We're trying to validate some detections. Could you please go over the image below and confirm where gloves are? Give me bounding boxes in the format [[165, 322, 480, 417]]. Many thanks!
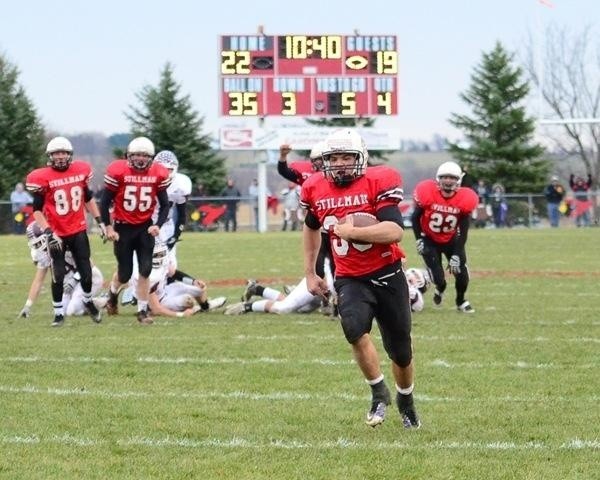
[[43, 231, 65, 256], [98, 222, 108, 244], [415, 238, 432, 257], [445, 254, 463, 276]]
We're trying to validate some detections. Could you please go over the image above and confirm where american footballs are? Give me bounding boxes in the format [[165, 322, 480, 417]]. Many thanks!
[[338, 213, 380, 227]]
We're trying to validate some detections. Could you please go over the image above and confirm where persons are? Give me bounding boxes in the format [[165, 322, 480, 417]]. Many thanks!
[[25, 136, 109, 328], [98, 135, 172, 325], [11, 183, 108, 318], [276, 140, 333, 279], [410, 161, 479, 315], [121, 269, 226, 318], [190, 177, 303, 232], [223, 258, 433, 318], [543, 173, 594, 228], [297, 127, 423, 430], [148, 149, 193, 278], [468, 177, 509, 229]]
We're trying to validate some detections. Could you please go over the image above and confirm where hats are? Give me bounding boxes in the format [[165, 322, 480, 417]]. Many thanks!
[[319, 128, 369, 179]]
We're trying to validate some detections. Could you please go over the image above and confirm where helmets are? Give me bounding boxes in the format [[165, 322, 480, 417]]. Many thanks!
[[434, 161, 465, 194], [45, 136, 74, 171], [406, 263, 430, 313], [24, 221, 48, 262], [153, 149, 179, 186], [308, 145, 321, 161], [125, 135, 157, 172], [150, 238, 170, 267]]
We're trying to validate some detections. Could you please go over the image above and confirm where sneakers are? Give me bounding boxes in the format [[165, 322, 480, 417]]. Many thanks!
[[282, 283, 295, 294], [240, 279, 258, 303], [432, 287, 443, 307], [81, 299, 101, 323], [222, 301, 247, 315], [395, 390, 421, 431], [135, 309, 153, 326], [48, 313, 65, 326], [17, 306, 30, 320], [106, 292, 119, 315], [364, 380, 392, 428], [456, 300, 475, 314], [202, 296, 227, 313]]
